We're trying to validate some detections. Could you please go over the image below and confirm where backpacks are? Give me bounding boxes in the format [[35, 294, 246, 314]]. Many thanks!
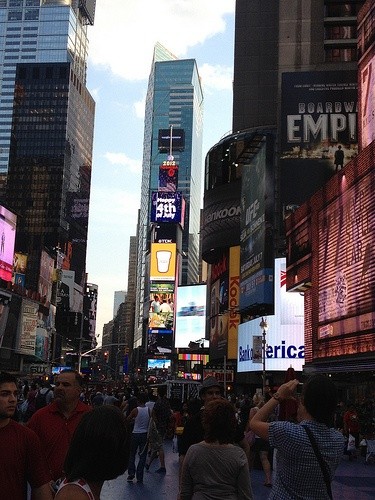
[[36, 388, 51, 409]]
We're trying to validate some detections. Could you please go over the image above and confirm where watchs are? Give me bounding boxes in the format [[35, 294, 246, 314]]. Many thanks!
[[273, 393, 281, 402]]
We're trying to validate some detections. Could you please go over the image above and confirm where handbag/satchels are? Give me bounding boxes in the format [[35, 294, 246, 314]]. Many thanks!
[[250, 433, 262, 454], [21, 398, 30, 416]]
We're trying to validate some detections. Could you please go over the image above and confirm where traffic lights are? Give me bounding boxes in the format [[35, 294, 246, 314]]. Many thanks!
[[104, 353, 108, 360], [45, 376, 49, 382], [125, 347, 130, 354]]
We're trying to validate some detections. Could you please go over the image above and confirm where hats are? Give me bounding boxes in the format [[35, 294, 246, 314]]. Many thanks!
[[198, 376, 223, 398]]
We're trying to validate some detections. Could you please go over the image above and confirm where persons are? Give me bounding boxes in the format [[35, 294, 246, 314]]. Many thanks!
[[144, 387, 176, 473], [249, 373, 346, 500], [11, 375, 55, 424], [335, 398, 360, 454], [334, 144, 344, 171], [55, 406, 129, 500], [159, 298, 174, 312], [80, 374, 174, 415], [228, 392, 281, 487], [151, 295, 161, 312], [25, 369, 91, 497], [126, 395, 154, 482], [1, 232, 5, 255], [178, 375, 251, 471], [177, 400, 254, 500], [51, 269, 73, 310], [0, 370, 53, 500]]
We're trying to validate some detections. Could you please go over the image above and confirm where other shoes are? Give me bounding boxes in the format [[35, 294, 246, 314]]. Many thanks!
[[127, 475, 135, 482], [264, 483, 273, 488], [144, 463, 150, 470], [157, 467, 166, 474], [137, 473, 144, 482]]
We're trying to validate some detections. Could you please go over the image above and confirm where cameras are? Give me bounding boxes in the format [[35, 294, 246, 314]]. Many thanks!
[[294, 383, 304, 395]]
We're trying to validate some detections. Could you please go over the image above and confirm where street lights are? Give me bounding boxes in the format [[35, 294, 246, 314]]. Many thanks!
[[259, 317, 270, 396]]
